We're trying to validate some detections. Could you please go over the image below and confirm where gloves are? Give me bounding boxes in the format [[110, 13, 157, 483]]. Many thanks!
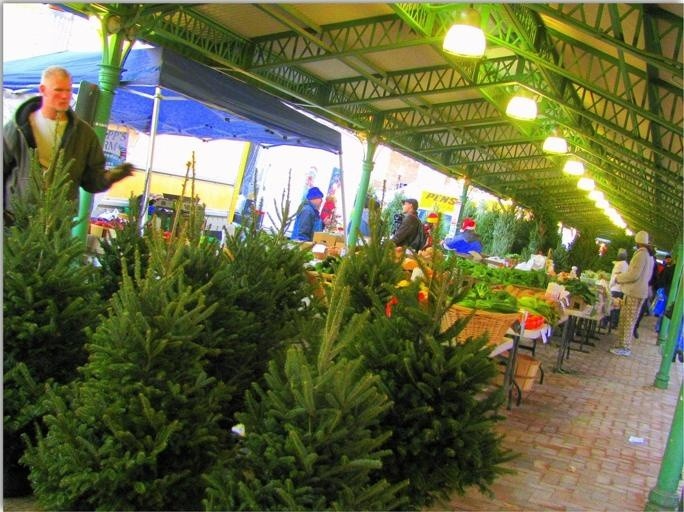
[[107, 164, 134, 184]]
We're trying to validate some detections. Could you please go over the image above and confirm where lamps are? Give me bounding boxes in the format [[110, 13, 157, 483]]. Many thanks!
[[438, 4, 595, 191]]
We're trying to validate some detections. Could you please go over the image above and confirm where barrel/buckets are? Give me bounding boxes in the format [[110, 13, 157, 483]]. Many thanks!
[[487, 353, 542, 399]]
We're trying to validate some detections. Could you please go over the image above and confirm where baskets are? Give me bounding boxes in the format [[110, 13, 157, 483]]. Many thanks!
[[508, 284, 547, 300], [487, 283, 507, 293], [442, 299, 524, 348]]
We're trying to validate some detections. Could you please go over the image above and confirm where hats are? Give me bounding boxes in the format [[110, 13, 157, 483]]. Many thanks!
[[401, 198, 418, 211], [460, 217, 475, 233], [617, 248, 627, 259], [306, 187, 323, 200], [635, 230, 648, 245]]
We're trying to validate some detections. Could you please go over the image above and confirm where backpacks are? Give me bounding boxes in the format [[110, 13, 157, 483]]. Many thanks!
[[409, 216, 427, 251]]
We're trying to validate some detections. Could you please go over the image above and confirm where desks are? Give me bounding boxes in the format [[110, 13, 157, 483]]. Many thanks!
[[436, 294, 622, 411]]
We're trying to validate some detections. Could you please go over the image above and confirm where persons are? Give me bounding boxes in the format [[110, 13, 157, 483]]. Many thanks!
[[391, 198, 424, 253], [347, 200, 382, 247], [290, 186, 325, 241], [2, 63, 135, 226], [532, 248, 547, 270], [442, 227, 482, 254], [600, 230, 675, 357]]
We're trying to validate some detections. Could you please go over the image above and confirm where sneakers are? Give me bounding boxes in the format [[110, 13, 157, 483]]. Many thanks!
[[610, 344, 631, 358]]
